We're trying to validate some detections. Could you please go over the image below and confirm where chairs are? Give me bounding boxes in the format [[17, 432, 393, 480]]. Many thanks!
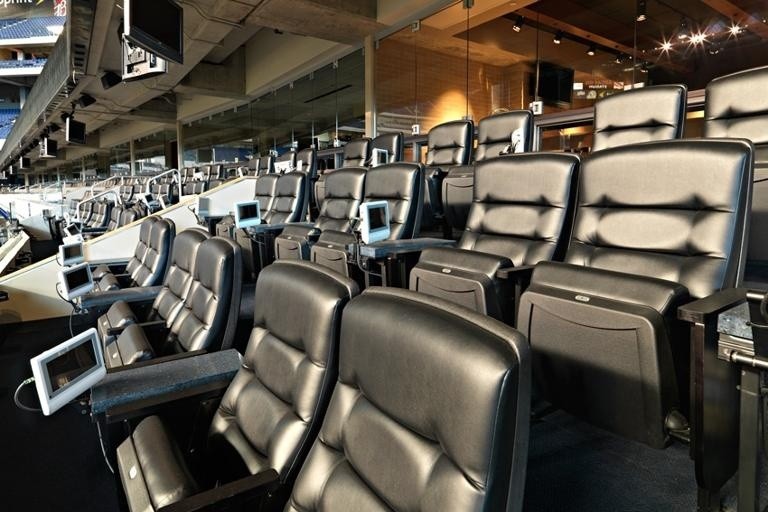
[[281, 152, 296, 172], [296, 148, 318, 180], [367, 130, 404, 166], [341, 137, 373, 167], [95, 214, 163, 281], [115, 257, 361, 511], [99, 218, 177, 291], [217, 173, 279, 240], [426, 120, 475, 166], [68, 160, 236, 230], [518, 136, 755, 512], [95, 226, 211, 345], [408, 153, 582, 331], [267, 166, 368, 263], [259, 154, 274, 176], [704, 65, 768, 262], [310, 160, 426, 285], [248, 158, 260, 175], [167, 283, 534, 512], [106, 234, 243, 376], [473, 109, 534, 161], [590, 84, 687, 152], [233, 170, 310, 277]]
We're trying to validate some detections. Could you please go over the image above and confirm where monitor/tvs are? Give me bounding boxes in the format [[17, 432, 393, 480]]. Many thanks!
[[44, 138, 58, 159], [537, 60, 574, 104], [197, 197, 211, 215], [31, 328, 106, 416], [66, 117, 86, 144], [62, 262, 94, 301], [373, 149, 389, 165], [124, 0, 184, 67], [235, 201, 261, 228], [360, 203, 390, 243], [144, 194, 154, 202], [65, 223, 80, 235], [121, 33, 168, 82], [61, 243, 82, 264]]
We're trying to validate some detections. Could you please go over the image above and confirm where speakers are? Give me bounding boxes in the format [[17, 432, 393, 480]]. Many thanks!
[[78, 94, 96, 108], [101, 73, 121, 89]]
